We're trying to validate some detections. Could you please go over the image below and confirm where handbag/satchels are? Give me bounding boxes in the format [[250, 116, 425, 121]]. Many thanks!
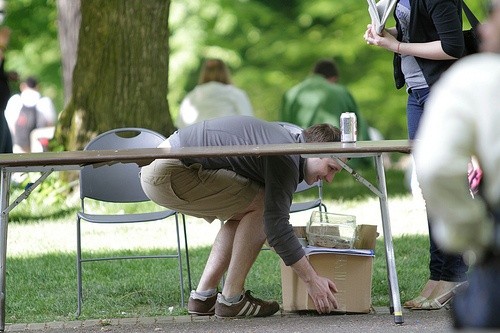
[[462, 0, 482, 57]]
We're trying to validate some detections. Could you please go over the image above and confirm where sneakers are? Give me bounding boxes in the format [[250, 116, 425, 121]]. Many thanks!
[[188, 289, 280, 319]]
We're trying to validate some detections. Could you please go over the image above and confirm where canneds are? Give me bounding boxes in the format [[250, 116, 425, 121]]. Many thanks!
[[339, 112, 357, 142]]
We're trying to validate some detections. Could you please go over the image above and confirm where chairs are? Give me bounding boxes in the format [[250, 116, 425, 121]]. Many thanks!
[[220, 121, 329, 288], [75, 129, 192, 316]]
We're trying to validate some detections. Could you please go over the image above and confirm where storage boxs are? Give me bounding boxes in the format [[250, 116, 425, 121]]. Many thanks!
[[265, 225, 380, 313]]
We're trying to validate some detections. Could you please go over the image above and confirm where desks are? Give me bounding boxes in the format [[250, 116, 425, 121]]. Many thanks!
[[0, 139, 412, 333]]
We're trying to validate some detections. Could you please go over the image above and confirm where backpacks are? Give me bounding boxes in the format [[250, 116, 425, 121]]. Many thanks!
[[15, 103, 38, 152]]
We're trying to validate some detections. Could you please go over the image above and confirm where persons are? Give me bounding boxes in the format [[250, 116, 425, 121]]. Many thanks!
[[363, 0, 468, 310], [138, 115, 338, 319], [4, 77, 58, 192], [411, 0, 500, 333], [176, 58, 253, 128], [279, 61, 390, 169], [0, 25, 13, 154]]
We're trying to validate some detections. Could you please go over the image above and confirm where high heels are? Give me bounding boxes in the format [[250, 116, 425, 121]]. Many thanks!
[[402, 281, 469, 311]]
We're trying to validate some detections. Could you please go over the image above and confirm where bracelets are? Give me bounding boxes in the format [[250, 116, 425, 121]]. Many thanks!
[[398, 42, 401, 55]]
